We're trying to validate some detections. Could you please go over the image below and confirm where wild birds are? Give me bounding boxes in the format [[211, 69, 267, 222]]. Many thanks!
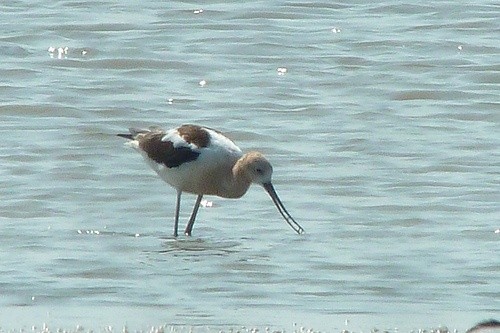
[[115, 123, 306, 239]]
